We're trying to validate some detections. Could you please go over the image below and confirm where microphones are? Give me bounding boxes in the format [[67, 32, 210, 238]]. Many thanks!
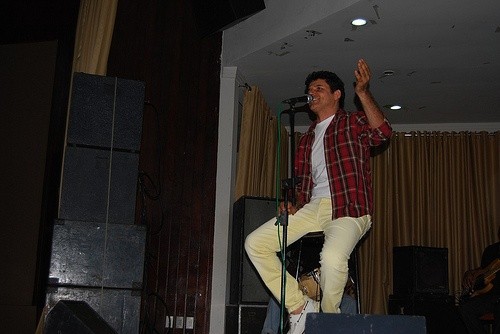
[[283, 94, 313, 104]]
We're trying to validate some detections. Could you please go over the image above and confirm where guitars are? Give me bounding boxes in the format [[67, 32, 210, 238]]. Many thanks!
[[461, 258, 500, 303]]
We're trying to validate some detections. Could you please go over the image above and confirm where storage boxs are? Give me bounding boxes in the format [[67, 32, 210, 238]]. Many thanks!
[[225, 304, 269, 334]]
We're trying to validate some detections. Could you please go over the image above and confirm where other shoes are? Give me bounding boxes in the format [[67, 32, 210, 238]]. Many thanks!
[[286, 299, 318, 334]]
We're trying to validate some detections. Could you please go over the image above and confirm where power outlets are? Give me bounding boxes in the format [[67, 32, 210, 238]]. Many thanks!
[[166, 315, 194, 329]]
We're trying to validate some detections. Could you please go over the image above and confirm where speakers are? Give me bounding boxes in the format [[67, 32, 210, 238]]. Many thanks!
[[44, 300, 116, 334]]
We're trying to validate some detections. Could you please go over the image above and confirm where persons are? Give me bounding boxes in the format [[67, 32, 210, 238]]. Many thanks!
[[244, 58, 392, 334], [457, 229, 500, 334]]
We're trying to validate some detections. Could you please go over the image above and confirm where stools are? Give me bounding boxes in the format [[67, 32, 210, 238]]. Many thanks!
[[480, 311, 495, 334], [295, 231, 360, 314]]
